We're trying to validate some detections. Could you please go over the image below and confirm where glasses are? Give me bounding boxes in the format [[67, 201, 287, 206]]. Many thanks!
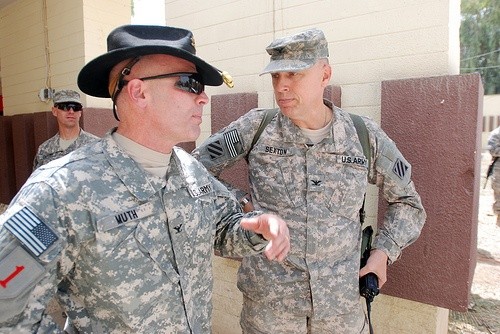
[[55, 104, 83, 112], [123, 72, 205, 96]]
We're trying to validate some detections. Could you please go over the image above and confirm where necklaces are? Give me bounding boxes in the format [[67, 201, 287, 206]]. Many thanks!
[[323, 106, 327, 128]]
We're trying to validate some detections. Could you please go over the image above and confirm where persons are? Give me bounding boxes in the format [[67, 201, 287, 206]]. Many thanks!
[[489, 117, 500, 226], [189, 27, 427, 334], [34, 90, 102, 172], [0, 24, 292, 334]]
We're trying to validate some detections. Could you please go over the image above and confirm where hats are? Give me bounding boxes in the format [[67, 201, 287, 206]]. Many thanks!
[[259, 28, 330, 75], [52, 90, 82, 106], [77, 25, 235, 98]]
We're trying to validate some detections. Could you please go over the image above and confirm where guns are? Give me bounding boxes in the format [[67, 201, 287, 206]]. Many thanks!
[[483, 157, 499, 190], [361, 225, 378, 301]]
